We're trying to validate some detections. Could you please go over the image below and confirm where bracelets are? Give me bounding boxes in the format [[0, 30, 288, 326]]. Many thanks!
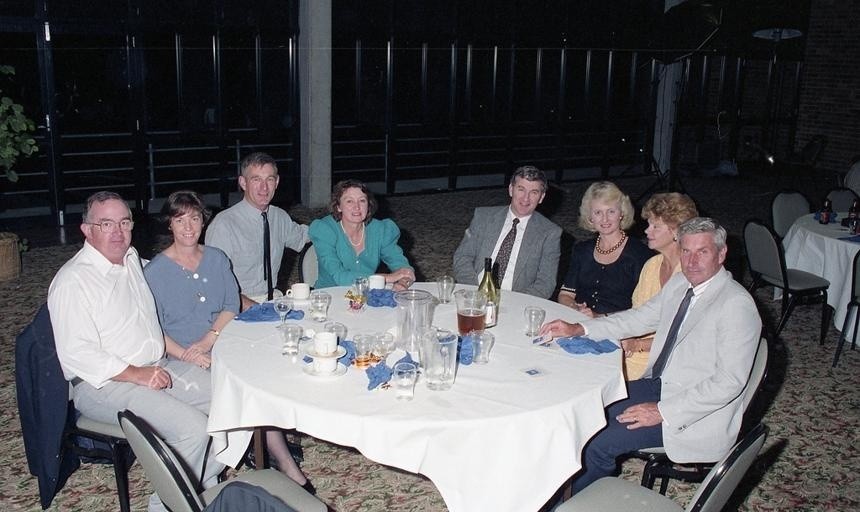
[[206, 328, 221, 336], [636, 337, 646, 354]]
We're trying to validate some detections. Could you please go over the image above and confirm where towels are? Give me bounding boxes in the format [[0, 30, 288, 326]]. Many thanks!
[[556, 336, 620, 355], [336, 338, 357, 366], [365, 288, 400, 307], [234, 302, 305, 321], [814, 209, 837, 224], [365, 350, 418, 391], [458, 335, 476, 365]]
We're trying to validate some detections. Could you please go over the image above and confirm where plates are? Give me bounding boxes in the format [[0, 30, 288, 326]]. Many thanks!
[[282, 296, 315, 306], [304, 342, 347, 359], [303, 362, 347, 377], [366, 283, 394, 289]]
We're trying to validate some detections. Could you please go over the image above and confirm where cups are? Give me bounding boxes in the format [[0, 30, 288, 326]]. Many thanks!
[[354, 333, 374, 368], [279, 323, 300, 355], [391, 289, 434, 352], [373, 330, 392, 360], [422, 328, 458, 391], [471, 330, 494, 365], [368, 275, 386, 290], [309, 289, 332, 323], [394, 362, 416, 401], [524, 306, 546, 337], [324, 322, 346, 341], [453, 287, 488, 336], [312, 358, 337, 373], [314, 332, 337, 356], [352, 278, 368, 298], [273, 297, 294, 328], [285, 282, 312, 299], [436, 275, 453, 304]]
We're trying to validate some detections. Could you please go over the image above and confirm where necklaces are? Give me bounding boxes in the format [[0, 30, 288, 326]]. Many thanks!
[[340, 220, 365, 247], [595, 232, 626, 255]]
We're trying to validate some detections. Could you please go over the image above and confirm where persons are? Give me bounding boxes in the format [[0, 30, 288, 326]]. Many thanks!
[[620, 193, 699, 382], [308, 180, 416, 296], [558, 180, 662, 317], [143, 191, 317, 495], [454, 166, 564, 300], [539, 217, 762, 500], [47, 191, 227, 512], [205, 150, 311, 314]]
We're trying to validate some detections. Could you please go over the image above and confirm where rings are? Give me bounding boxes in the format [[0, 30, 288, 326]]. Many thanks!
[[634, 417, 637, 423]]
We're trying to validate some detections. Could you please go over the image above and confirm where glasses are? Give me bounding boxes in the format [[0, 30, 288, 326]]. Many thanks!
[[173, 218, 201, 225], [81, 220, 134, 234]]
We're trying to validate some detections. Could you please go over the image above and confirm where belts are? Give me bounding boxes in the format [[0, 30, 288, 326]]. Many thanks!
[[69, 375, 83, 389]]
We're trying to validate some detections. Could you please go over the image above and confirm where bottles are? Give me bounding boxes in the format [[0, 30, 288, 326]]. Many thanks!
[[849, 198, 860, 226], [819, 199, 831, 225], [478, 257, 500, 328]]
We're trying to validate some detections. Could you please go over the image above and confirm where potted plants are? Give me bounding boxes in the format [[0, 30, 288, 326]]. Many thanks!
[[0, 97, 40, 284]]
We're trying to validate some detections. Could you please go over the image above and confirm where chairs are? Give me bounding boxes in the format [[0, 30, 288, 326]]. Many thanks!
[[299, 239, 320, 289], [831, 252, 860, 367], [637, 335, 789, 496], [742, 220, 831, 350], [554, 422, 770, 511], [821, 187, 860, 214], [30, 301, 136, 512], [117, 408, 326, 512], [772, 192, 812, 241]]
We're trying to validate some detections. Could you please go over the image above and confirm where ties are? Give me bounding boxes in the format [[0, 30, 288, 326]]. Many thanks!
[[491, 218, 521, 291], [651, 289, 695, 381], [261, 212, 274, 301]]
